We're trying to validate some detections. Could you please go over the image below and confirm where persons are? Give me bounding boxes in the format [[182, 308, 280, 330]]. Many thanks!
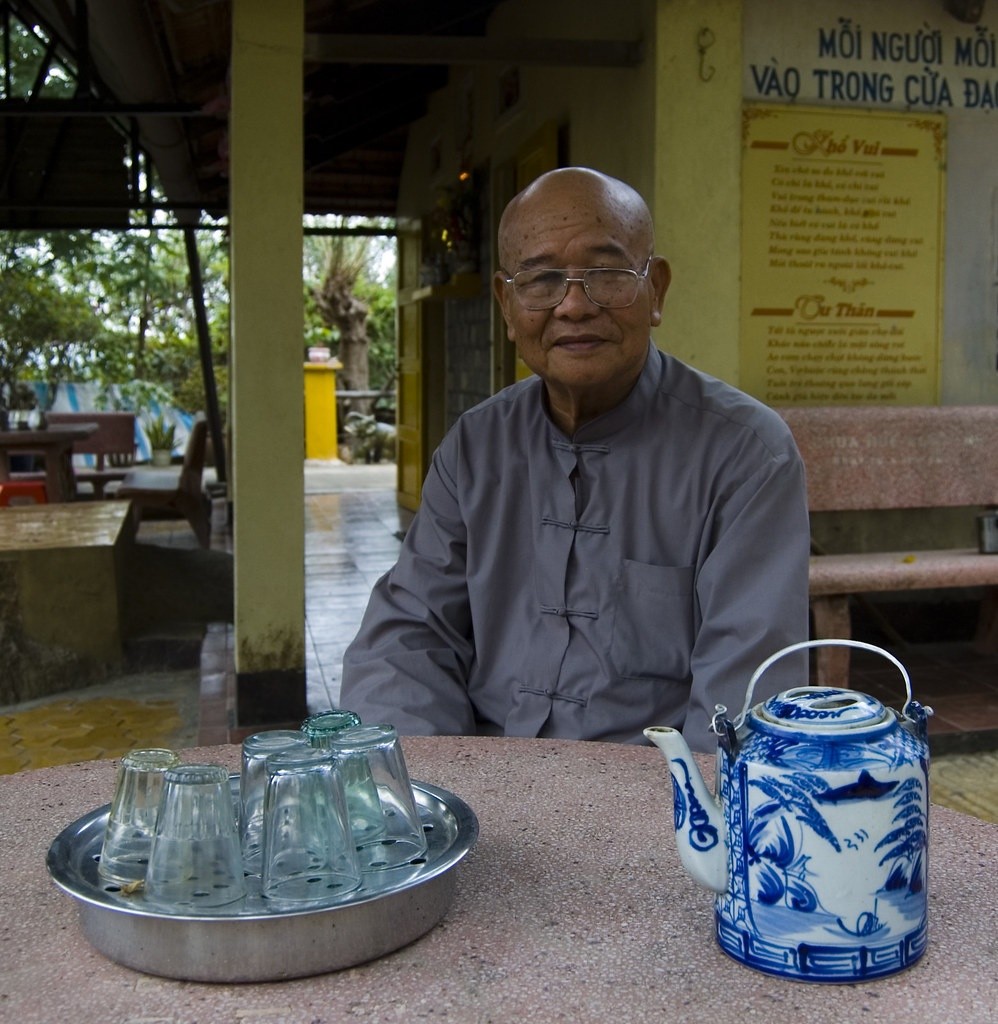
[[340, 168, 810, 755]]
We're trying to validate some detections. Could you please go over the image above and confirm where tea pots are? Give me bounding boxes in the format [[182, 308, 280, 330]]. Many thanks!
[[643, 638, 935, 985]]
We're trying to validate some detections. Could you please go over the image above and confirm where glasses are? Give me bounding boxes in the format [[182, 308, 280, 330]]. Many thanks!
[[501, 253, 652, 312]]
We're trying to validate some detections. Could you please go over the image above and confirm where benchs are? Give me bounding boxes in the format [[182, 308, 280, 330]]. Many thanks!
[[43, 411, 135, 471], [116, 420, 213, 541], [767, 405, 998, 690]]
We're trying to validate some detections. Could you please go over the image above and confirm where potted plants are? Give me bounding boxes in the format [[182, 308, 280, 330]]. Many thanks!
[[142, 413, 182, 468]]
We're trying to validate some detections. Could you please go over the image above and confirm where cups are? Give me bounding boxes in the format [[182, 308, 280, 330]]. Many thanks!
[[97, 746, 183, 888], [238, 728, 313, 878], [330, 723, 428, 873], [299, 710, 387, 848], [258, 745, 362, 903], [142, 763, 248, 911]]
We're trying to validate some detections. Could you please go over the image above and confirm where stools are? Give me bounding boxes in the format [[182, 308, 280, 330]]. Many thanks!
[[0, 478, 50, 509]]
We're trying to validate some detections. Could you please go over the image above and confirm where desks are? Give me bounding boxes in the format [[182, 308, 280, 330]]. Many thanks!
[[336, 391, 395, 424], [0, 500, 137, 706], [1, 736, 998, 1023], [0, 421, 99, 505]]
